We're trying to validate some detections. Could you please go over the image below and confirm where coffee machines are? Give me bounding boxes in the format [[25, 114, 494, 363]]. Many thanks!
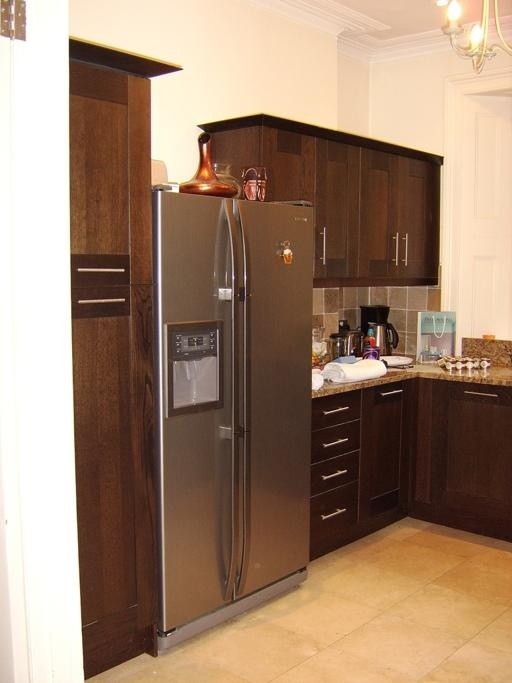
[[167, 322, 227, 420]]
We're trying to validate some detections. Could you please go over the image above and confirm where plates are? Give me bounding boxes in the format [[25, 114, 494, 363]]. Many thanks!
[[377, 355, 414, 367]]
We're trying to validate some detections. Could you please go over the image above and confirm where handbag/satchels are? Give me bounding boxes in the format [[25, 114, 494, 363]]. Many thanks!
[[415, 311, 456, 364]]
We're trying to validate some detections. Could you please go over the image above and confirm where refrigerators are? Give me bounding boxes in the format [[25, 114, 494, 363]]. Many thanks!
[[154, 188, 325, 651]]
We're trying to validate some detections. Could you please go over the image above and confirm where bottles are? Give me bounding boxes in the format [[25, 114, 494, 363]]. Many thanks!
[[178, 130, 240, 199]]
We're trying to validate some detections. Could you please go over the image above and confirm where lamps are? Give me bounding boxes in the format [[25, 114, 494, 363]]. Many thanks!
[[436, 0, 512, 74]]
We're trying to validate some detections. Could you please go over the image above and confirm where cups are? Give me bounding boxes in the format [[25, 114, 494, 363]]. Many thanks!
[[440, 356, 491, 371], [366, 319, 399, 356]]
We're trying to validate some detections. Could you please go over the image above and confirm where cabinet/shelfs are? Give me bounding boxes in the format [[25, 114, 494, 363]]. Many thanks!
[[362, 137, 444, 287], [309, 377, 512, 566], [197, 114, 361, 287], [70, 36, 184, 679]]
